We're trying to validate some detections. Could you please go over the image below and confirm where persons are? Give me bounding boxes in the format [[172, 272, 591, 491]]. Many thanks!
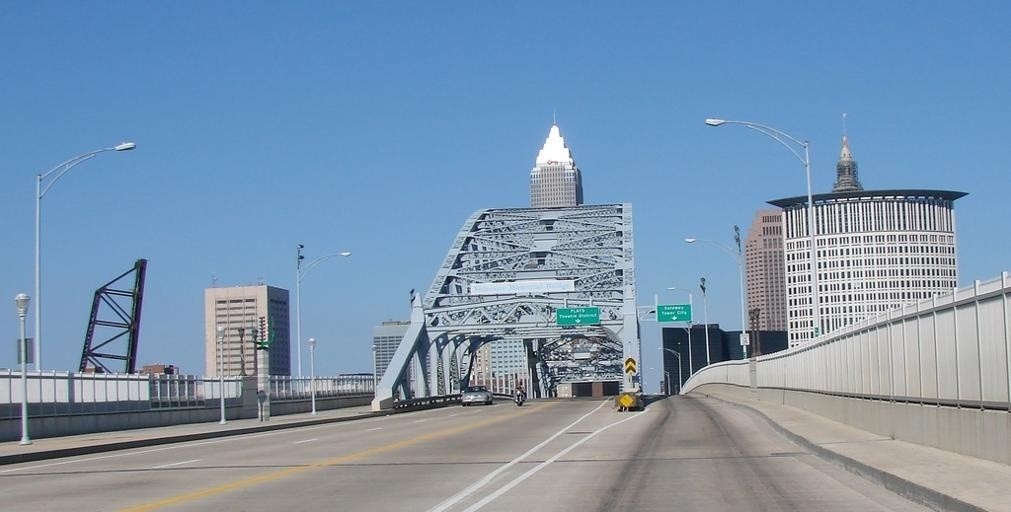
[[515, 383, 527, 401]]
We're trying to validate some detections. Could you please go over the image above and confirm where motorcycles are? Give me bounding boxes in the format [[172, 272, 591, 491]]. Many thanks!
[[512, 388, 527, 406]]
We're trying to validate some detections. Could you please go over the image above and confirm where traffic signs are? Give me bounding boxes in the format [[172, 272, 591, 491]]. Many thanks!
[[557, 306, 600, 325], [624, 358, 636, 374], [657, 303, 693, 322]]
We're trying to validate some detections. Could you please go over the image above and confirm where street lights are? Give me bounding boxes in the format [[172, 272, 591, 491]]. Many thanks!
[[656, 346, 682, 391], [12, 292, 33, 449], [217, 325, 229, 425], [251, 326, 259, 376], [666, 286, 694, 379], [372, 344, 380, 399], [32, 140, 139, 373], [238, 326, 248, 376], [685, 237, 749, 358], [650, 367, 672, 395], [297, 242, 353, 393], [753, 307, 762, 356], [308, 337, 319, 416], [747, 309, 756, 356], [704, 117, 821, 337]]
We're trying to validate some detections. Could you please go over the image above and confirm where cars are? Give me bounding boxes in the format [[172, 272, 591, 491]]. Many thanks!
[[461, 385, 493, 406]]
